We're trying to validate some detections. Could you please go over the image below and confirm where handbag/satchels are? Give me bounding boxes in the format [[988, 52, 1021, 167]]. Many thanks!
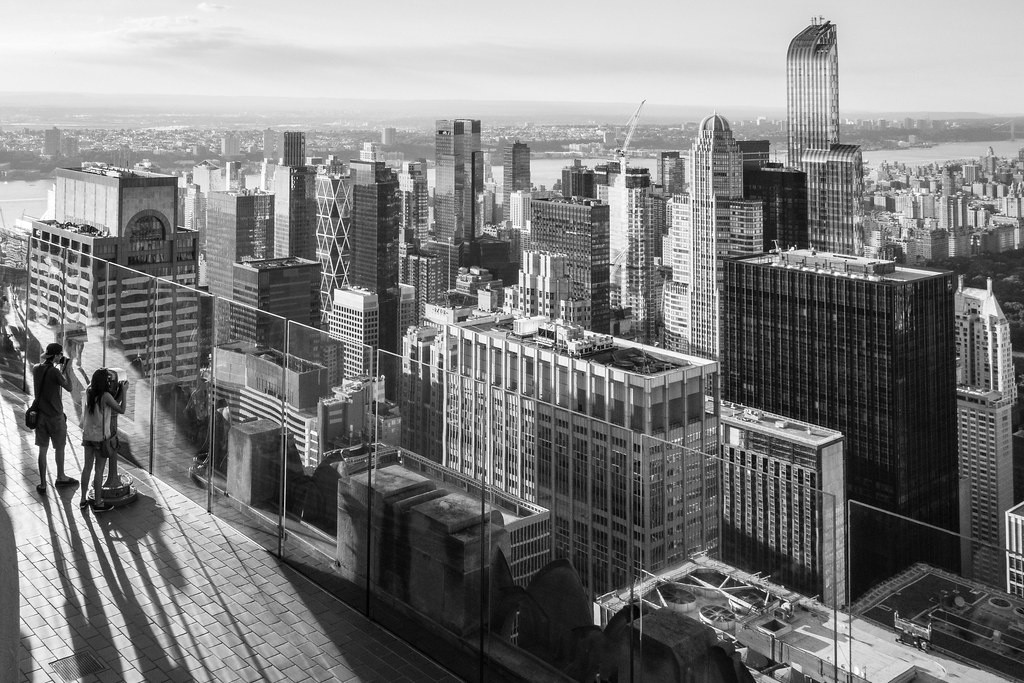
[[101, 435, 119, 458], [25, 406, 39, 429]]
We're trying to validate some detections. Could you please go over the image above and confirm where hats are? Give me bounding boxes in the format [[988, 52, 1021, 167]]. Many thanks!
[[41, 344, 63, 359]]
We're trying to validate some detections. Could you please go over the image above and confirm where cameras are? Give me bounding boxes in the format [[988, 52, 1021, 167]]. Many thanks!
[[59, 356, 70, 365]]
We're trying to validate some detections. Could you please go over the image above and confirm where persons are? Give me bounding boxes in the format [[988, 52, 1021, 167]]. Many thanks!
[[32, 343, 78, 493], [79, 368, 128, 511]]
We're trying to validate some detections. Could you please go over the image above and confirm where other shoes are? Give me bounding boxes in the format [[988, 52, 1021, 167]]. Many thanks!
[[55, 478, 79, 487], [93, 503, 114, 512], [37, 484, 46, 494], [80, 500, 89, 508]]
